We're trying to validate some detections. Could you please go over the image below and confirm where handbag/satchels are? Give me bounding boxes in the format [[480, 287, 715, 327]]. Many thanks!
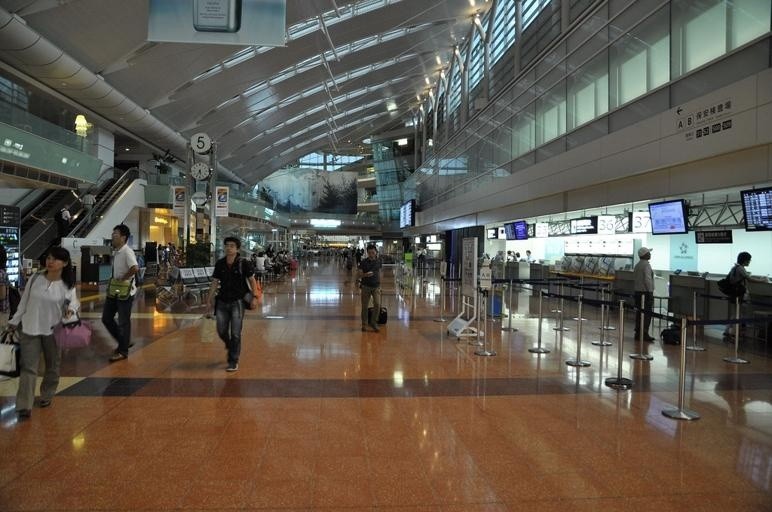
[[661, 325, 682, 344], [717, 278, 746, 299], [107, 277, 133, 300], [244, 276, 261, 311], [0, 329, 21, 377], [52, 307, 91, 349]]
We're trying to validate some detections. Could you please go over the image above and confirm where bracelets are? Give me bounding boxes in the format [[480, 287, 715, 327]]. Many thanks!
[[252, 295, 257, 298]]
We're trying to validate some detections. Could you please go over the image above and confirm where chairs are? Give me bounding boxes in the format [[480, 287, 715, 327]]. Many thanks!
[[173, 267, 214, 311]]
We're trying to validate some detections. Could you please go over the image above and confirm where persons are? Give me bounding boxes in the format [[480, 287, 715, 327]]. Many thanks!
[[507, 250, 535, 262], [81, 191, 96, 224], [5, 247, 82, 418], [57, 203, 72, 241], [356, 245, 384, 332], [249, 243, 292, 279], [417, 247, 427, 267], [634, 247, 656, 341], [158, 243, 183, 268], [302, 244, 364, 269], [207, 237, 258, 372], [722, 252, 768, 345], [102, 225, 139, 362]]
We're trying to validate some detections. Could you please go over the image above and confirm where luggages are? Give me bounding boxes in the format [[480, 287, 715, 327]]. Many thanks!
[[368, 289, 387, 325]]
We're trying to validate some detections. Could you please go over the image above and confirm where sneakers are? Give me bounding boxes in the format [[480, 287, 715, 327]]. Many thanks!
[[226, 347, 239, 371], [362, 324, 380, 333], [722, 333, 741, 340], [109, 342, 133, 360]]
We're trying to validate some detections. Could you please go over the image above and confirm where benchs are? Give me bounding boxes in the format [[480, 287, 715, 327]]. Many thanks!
[[253, 268, 275, 281]]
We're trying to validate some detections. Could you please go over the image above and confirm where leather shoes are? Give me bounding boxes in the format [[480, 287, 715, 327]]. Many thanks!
[[20, 400, 51, 419], [635, 333, 655, 341]]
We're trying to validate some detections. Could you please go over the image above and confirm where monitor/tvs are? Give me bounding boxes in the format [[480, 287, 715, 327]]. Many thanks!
[[648, 199, 688, 235], [740, 186, 772, 232], [504, 222, 516, 240], [513, 220, 528, 240]]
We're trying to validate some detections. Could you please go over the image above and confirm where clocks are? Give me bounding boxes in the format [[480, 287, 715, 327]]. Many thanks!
[[189, 163, 209, 181]]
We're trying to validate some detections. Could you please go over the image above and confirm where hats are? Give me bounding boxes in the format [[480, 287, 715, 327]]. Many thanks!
[[638, 248, 652, 256]]
